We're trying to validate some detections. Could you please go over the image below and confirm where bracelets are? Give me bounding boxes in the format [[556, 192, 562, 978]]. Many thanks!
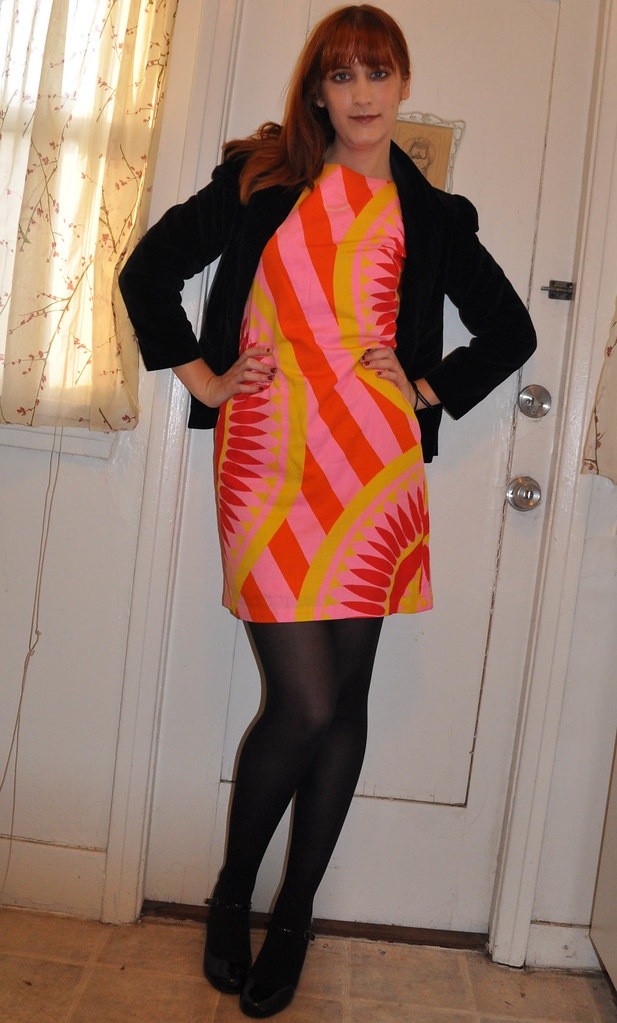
[[410, 381, 431, 411]]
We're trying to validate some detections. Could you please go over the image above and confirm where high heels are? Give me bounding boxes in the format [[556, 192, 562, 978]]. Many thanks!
[[203, 883, 254, 994], [239, 913, 316, 1018]]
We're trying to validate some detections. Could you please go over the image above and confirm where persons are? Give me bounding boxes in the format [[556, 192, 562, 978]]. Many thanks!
[[118, 4, 538, 1019]]
